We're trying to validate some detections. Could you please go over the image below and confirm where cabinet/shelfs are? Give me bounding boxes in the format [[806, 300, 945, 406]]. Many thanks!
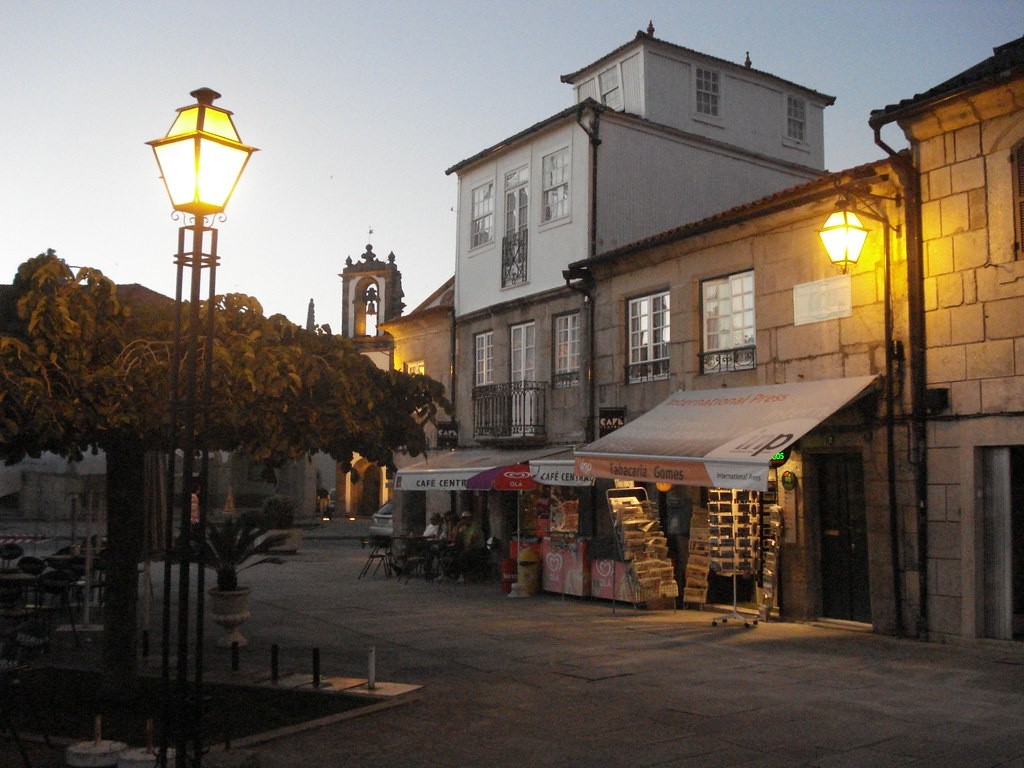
[[605, 487, 678, 614]]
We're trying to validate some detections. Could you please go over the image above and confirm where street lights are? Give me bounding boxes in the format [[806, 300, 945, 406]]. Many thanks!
[[145, 87, 262, 768]]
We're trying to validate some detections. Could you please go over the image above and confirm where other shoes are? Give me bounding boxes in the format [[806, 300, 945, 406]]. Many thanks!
[[438, 574, 450, 585]]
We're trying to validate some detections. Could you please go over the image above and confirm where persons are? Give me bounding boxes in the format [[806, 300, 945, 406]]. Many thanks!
[[392, 510, 485, 584], [316, 484, 331, 516]]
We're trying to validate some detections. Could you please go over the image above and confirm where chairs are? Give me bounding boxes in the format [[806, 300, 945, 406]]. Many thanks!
[[0, 537, 104, 654], [358, 538, 478, 584]]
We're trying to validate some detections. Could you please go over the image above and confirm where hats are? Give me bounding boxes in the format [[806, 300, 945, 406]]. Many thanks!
[[459, 511, 472, 518]]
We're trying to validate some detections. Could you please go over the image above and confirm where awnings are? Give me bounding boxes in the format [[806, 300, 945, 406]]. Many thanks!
[[393, 447, 578, 490], [528, 441, 597, 486], [574, 376, 882, 493]]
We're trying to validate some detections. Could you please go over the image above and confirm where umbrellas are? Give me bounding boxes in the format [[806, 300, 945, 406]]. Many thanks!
[[467, 461, 544, 582]]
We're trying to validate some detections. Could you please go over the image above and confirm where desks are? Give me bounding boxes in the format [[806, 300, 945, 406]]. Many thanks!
[[392, 536, 436, 539], [590, 554, 637, 603], [509, 536, 542, 593], [541, 537, 590, 597]]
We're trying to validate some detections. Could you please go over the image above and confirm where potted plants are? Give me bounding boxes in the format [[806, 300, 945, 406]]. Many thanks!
[[161, 508, 298, 648]]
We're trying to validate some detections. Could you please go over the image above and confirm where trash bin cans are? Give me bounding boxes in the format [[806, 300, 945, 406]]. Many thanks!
[[263, 492, 296, 530], [518, 547, 540, 595]]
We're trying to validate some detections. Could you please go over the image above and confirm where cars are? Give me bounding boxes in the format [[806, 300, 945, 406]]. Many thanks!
[[368, 499, 393, 544]]
